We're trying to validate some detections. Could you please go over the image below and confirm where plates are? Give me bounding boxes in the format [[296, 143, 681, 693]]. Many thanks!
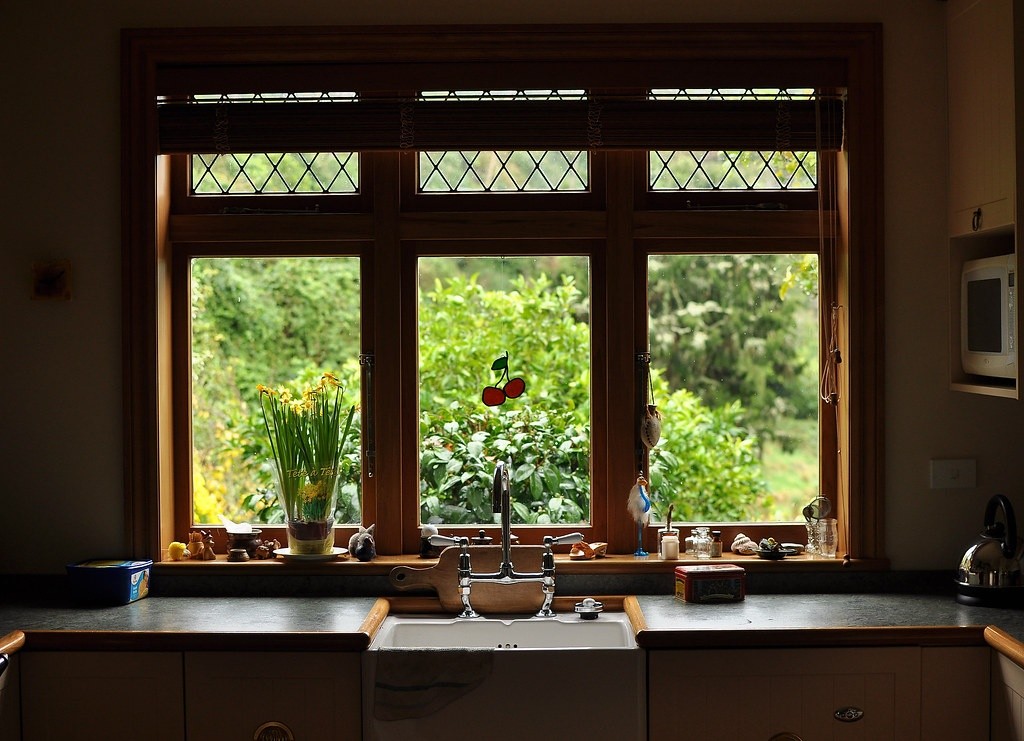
[[273, 547, 349, 561]]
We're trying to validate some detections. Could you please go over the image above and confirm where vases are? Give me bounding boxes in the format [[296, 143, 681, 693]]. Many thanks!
[[288, 519, 334, 554]]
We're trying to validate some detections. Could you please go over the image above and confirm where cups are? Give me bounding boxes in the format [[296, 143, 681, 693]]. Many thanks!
[[227, 529, 262, 558]]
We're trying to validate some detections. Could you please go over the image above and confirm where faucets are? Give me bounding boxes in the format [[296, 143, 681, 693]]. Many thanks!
[[492, 461, 513, 569]]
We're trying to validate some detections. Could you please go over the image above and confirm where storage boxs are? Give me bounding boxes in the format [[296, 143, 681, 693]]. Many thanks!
[[65, 558, 153, 604], [675, 565, 747, 605]]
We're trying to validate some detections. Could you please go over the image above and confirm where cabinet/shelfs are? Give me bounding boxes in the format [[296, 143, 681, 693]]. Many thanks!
[[18, 650, 364, 741], [945, 0, 1024, 400], [648, 647, 1024, 741]]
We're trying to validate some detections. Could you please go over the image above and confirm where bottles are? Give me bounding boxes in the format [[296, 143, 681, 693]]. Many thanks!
[[685, 527, 712, 558], [818, 518, 838, 558], [710, 530, 722, 558], [661, 532, 680, 560]]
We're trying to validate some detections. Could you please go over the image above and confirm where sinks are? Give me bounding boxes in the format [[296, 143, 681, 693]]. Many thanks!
[[369, 613, 641, 741]]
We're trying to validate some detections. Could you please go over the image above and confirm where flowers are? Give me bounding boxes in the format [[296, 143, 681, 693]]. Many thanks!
[[255, 374, 356, 520]]
